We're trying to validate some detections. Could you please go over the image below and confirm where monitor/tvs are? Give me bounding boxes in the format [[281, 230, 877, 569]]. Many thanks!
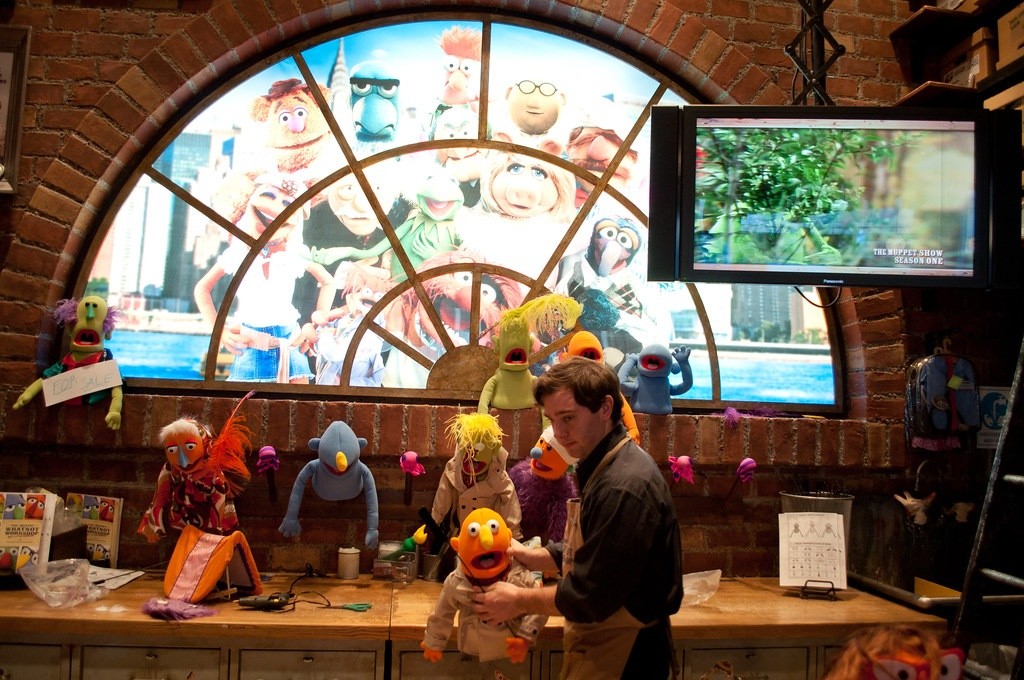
[[680, 105, 990, 289]]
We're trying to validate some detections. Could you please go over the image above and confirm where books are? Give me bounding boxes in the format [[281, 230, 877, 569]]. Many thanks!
[[65, 491, 125, 571], [0, 490, 57, 577]]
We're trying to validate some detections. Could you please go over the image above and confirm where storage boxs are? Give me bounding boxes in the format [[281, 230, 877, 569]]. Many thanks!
[[997, 1, 1024, 67], [924, 24, 993, 89]]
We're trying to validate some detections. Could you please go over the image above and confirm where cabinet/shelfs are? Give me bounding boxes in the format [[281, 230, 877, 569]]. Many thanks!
[[1, 570, 394, 680], [388, 574, 950, 679]]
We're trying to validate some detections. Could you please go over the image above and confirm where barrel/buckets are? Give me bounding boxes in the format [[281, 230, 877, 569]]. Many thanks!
[[780, 488, 854, 592]]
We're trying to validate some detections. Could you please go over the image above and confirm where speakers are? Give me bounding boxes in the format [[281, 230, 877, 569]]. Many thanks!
[[647, 104, 677, 281], [990, 108, 1022, 292]]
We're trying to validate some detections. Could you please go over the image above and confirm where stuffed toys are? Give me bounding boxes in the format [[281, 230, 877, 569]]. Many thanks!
[[505, 424, 587, 553], [280, 421, 381, 547], [8, 294, 126, 429], [418, 507, 546, 661], [614, 341, 694, 417], [475, 292, 582, 433], [139, 413, 241, 549], [431, 409, 526, 543], [826, 624, 963, 680]]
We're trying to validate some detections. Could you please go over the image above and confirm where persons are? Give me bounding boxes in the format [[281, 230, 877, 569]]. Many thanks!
[[469, 358, 685, 680]]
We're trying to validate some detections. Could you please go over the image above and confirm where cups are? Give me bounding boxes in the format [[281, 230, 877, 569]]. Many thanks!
[[337, 547, 361, 579], [421, 551, 443, 582]]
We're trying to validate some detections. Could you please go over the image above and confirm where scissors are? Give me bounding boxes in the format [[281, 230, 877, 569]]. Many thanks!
[[317, 603, 372, 612]]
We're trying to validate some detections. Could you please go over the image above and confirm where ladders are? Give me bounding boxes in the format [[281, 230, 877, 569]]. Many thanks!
[[955, 336, 1024, 680]]
